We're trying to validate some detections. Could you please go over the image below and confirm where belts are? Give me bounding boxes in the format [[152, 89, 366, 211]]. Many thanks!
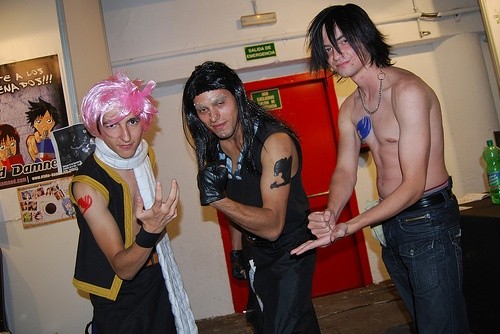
[[402, 188, 452, 214], [143, 253, 158, 268], [245, 233, 269, 243]]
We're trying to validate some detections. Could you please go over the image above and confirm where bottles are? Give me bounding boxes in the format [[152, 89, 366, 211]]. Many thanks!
[[482, 139, 500, 205]]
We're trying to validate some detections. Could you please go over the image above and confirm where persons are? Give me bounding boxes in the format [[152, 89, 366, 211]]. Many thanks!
[[181, 61, 320, 334], [67, 71, 199, 334], [290, 3, 471, 334]]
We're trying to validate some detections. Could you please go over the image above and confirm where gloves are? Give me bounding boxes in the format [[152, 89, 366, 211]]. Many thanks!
[[197, 160, 229, 206], [230, 250, 250, 281]]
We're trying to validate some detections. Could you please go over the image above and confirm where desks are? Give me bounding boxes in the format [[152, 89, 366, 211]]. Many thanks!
[[460, 197, 500, 334]]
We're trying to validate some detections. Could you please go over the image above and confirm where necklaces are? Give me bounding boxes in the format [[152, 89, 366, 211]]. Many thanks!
[[357, 65, 385, 115]]
